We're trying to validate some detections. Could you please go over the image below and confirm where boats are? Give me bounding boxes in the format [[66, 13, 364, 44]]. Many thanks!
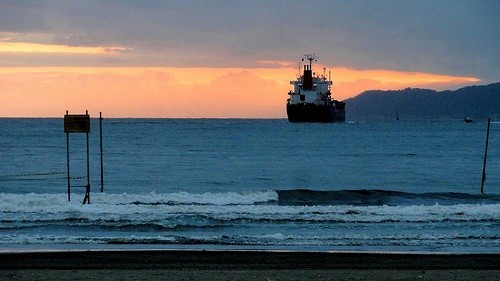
[[287, 52, 348, 124], [464, 117, 475, 125]]
